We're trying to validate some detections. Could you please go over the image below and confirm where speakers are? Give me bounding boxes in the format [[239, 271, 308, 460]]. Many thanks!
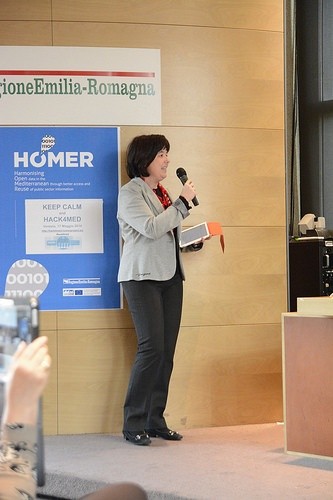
[[290, 238, 333, 312]]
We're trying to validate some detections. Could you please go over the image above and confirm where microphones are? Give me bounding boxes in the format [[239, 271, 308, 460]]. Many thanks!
[[176, 168, 199, 206]]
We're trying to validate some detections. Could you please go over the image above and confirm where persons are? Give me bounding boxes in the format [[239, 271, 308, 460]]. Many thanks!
[[117, 135, 212, 446], [1, 336, 149, 500]]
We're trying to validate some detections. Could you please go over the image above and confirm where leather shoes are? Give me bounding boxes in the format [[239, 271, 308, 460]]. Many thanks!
[[149, 428, 182, 440], [121, 430, 151, 445]]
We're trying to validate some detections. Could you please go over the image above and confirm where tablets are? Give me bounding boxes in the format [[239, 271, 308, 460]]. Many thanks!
[[179, 222, 210, 248]]
